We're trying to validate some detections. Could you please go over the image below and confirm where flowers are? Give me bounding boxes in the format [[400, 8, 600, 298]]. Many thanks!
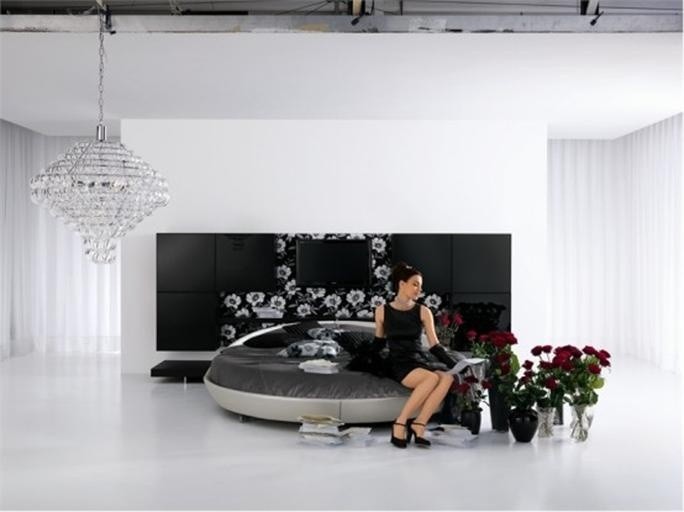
[[438, 313, 611, 405]]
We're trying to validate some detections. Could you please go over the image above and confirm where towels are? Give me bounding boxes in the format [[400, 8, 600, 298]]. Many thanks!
[[297, 358, 341, 375]]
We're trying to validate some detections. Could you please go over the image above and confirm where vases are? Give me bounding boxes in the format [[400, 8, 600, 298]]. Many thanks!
[[462, 379, 591, 443]]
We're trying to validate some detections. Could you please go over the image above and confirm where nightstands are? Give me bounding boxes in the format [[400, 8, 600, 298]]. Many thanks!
[[150, 360, 214, 384]]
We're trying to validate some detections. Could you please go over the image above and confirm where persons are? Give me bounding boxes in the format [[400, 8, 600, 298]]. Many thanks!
[[345, 263, 473, 448]]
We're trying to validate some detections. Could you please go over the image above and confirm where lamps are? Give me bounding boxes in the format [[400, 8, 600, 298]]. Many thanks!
[[29, 0, 170, 262]]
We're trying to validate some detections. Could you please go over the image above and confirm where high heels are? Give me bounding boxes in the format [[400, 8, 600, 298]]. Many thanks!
[[406, 418, 431, 446], [391, 417, 408, 449]]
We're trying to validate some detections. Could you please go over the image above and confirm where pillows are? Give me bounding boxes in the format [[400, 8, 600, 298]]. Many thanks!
[[284, 319, 338, 340], [335, 333, 388, 356], [345, 346, 394, 378], [244, 327, 299, 348], [276, 340, 342, 358]]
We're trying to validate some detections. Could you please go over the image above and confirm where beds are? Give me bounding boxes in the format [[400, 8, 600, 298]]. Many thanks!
[[204, 320, 445, 425]]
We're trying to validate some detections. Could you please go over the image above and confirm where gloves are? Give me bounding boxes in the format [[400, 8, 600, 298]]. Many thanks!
[[344, 335, 386, 371], [429, 343, 468, 374]]
